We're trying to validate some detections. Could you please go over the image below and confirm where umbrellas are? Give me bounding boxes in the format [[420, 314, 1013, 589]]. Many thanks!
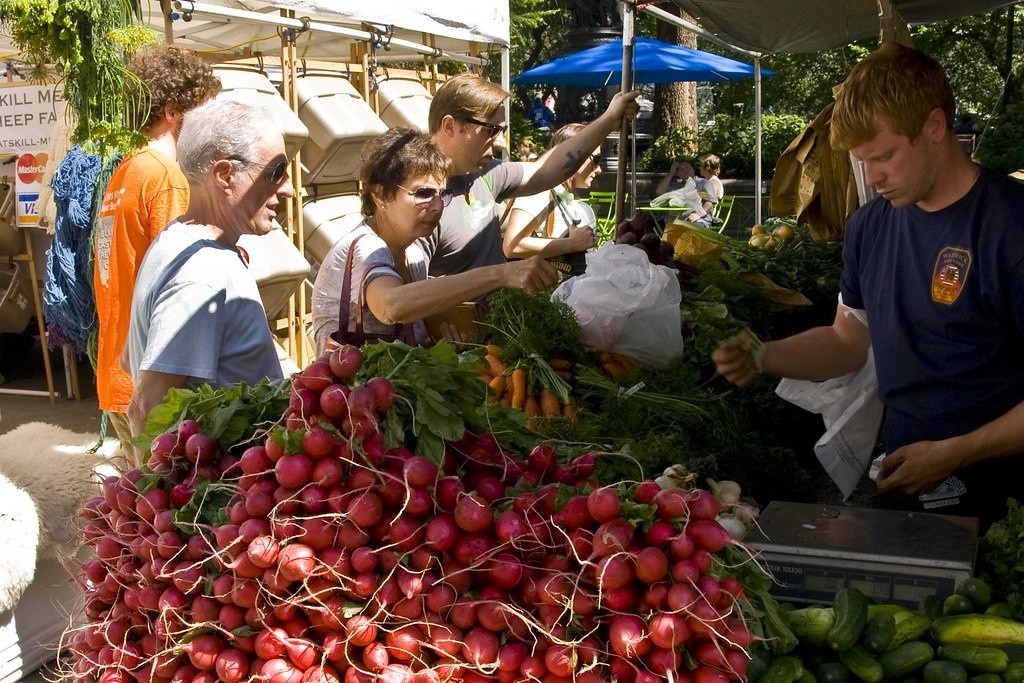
[[509, 31, 774, 225]]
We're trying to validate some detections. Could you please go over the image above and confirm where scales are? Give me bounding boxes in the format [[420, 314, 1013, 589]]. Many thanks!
[[739, 502, 981, 609]]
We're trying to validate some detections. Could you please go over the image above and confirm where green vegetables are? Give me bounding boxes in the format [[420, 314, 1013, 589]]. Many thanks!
[[581, 218, 843, 478]]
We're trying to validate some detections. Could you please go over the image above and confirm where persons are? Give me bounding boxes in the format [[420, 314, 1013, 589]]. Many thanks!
[[416, 76, 643, 353], [709, 45, 1023, 520], [117, 81, 292, 471], [684, 152, 725, 225], [308, 126, 560, 368], [656, 159, 715, 253], [92, 41, 220, 471], [500, 123, 603, 286]]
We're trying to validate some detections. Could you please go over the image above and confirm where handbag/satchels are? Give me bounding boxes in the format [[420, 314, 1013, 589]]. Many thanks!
[[651, 178, 708, 223], [324, 233, 418, 359], [551, 239, 685, 375], [773, 294, 883, 503], [495, 184, 586, 285]]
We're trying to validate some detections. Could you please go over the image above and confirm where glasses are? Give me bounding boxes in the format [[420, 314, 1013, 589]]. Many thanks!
[[462, 114, 511, 138], [396, 183, 455, 208], [589, 152, 604, 165], [226, 156, 289, 184]]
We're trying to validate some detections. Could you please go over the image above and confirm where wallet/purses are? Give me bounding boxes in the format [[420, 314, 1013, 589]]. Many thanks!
[[424, 301, 485, 346]]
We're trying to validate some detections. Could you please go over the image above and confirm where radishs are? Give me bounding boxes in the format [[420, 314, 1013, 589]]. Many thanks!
[[39, 339, 778, 683]]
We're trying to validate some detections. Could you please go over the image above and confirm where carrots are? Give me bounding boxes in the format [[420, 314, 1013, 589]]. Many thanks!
[[465, 286, 580, 431]]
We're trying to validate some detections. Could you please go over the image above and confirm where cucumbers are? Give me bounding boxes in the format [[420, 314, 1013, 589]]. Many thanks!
[[747, 578, 1024, 683]]
